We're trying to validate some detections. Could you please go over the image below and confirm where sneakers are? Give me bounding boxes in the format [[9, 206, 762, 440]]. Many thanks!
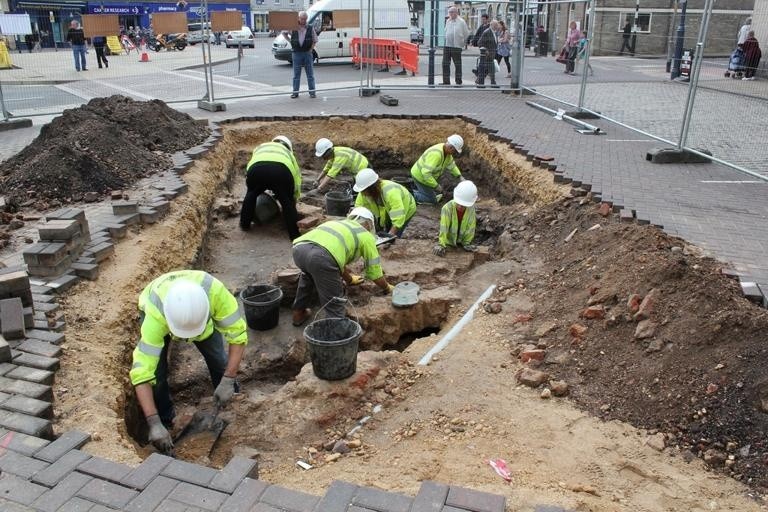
[[293, 308, 311, 325], [291, 94, 298, 98], [742, 77, 755, 80], [309, 93, 315, 98], [439, 67, 513, 88]]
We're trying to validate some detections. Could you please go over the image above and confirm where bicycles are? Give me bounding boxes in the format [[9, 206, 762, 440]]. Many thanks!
[[143, 34, 156, 51]]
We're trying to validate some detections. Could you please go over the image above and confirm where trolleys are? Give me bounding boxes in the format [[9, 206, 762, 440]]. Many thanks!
[[725, 46, 746, 79]]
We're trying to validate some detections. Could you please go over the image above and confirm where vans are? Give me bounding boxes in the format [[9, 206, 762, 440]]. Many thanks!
[[271, 0, 411, 66], [187, 23, 215, 45]]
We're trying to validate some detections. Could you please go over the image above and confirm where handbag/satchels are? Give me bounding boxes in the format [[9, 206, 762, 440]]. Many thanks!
[[556, 49, 569, 63]]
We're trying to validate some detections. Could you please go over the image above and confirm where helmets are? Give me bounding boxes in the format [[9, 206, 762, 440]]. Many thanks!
[[164, 280, 210, 338], [271, 135, 478, 234]]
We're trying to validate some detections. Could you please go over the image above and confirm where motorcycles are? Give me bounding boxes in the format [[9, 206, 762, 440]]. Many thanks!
[[156, 34, 188, 52]]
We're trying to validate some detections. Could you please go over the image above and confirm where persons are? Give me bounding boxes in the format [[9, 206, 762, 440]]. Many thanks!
[[313, 138, 373, 201], [239, 135, 303, 242], [292, 206, 395, 327], [411, 134, 464, 204], [433, 181, 479, 257], [14, 20, 153, 72], [321, 15, 332, 31], [129, 269, 248, 456], [618, 18, 631, 55], [213, 32, 222, 45], [737, 17, 751, 48], [438, 1, 594, 88], [353, 168, 416, 249], [282, 11, 318, 98], [742, 31, 761, 81]]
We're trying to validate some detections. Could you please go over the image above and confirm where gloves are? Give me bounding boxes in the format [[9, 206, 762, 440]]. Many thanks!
[[383, 283, 394, 293], [348, 276, 365, 286], [432, 244, 446, 256], [464, 244, 480, 252], [214, 376, 235, 408], [144, 414, 175, 453]]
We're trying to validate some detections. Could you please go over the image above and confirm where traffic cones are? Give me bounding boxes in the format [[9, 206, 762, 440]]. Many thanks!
[[0, 39, 12, 68]]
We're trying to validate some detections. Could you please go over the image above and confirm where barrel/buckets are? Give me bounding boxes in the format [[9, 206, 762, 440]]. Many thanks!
[[325, 191, 354, 216], [390, 176, 414, 197], [303, 296, 361, 380], [251, 190, 281, 225], [240, 284, 284, 330]]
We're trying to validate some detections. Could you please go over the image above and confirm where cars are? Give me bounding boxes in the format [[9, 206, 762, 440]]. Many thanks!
[[225, 26, 255, 48], [411, 28, 424, 42], [219, 32, 227, 42]]
